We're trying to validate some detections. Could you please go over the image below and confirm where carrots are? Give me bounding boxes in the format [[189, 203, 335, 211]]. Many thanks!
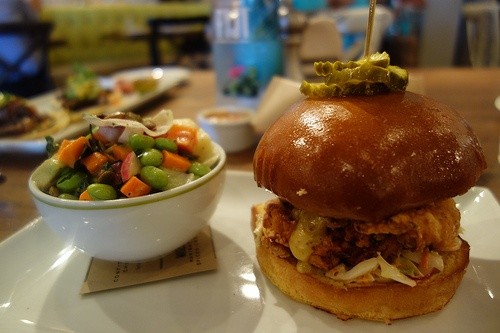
[[59, 136, 90, 170], [82, 152, 108, 174], [120, 176, 152, 198], [160, 127, 200, 171]]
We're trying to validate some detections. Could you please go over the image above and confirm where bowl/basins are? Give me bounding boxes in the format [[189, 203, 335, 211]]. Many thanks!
[[196, 106, 255, 154], [27, 132, 227, 263]]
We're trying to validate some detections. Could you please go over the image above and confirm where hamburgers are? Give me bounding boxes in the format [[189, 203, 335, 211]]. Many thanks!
[[251, 91, 486, 323]]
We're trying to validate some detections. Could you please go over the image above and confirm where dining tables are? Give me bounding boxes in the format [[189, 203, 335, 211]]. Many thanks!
[[0, 67, 500, 333]]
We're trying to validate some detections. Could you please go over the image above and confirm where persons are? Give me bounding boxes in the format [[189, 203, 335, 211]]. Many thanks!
[[0, 0, 47, 87], [287, 0, 369, 49]]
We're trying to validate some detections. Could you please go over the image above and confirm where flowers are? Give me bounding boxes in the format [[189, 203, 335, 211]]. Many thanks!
[[228, 63, 246, 76]]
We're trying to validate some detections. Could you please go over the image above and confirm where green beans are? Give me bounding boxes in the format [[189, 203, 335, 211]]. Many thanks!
[[52, 134, 211, 201]]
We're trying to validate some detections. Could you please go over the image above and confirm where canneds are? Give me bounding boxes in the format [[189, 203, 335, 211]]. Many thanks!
[[208, 0, 286, 108]]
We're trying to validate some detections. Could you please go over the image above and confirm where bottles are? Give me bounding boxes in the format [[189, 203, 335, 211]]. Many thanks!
[[211, 0, 285, 102]]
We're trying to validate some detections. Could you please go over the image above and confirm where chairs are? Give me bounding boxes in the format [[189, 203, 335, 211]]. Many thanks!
[[148, 16, 209, 68], [464, 3, 500, 69], [0, 19, 57, 93]]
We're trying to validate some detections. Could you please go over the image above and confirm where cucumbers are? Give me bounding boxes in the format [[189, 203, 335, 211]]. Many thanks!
[[299, 51, 410, 96]]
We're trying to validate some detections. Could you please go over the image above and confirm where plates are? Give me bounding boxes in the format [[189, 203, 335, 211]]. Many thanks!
[[0, 164, 499, 333], [0, 64, 190, 157]]
